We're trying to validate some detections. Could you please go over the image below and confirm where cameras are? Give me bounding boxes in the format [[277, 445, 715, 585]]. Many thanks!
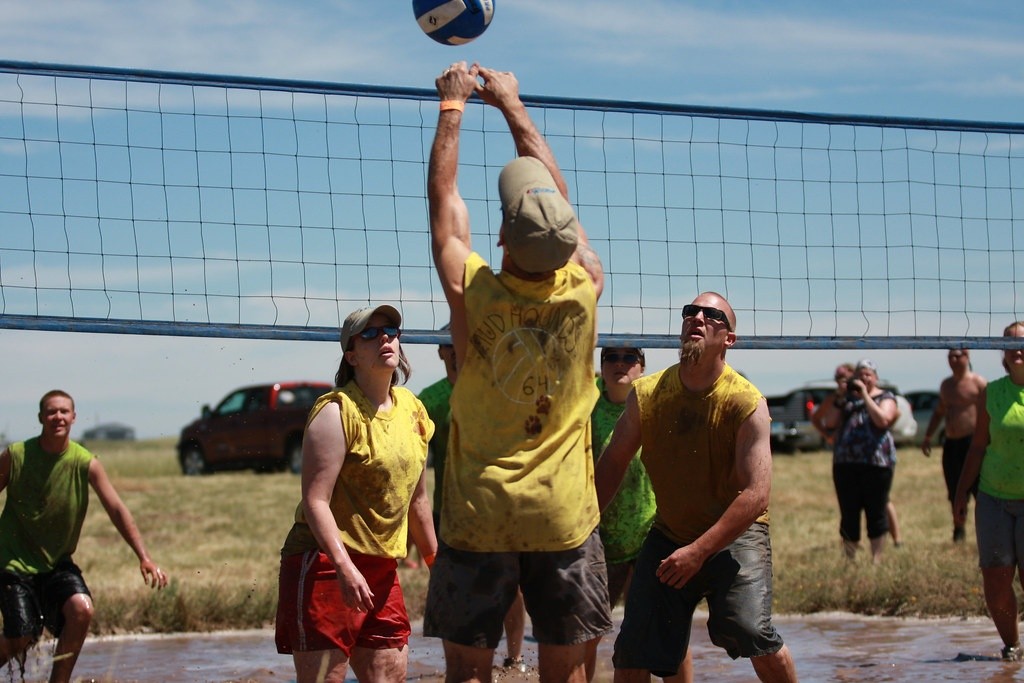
[[844, 378, 862, 392]]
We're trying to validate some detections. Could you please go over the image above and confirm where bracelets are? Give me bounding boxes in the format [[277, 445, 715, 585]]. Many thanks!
[[440, 100, 464, 113], [424, 552, 437, 566]]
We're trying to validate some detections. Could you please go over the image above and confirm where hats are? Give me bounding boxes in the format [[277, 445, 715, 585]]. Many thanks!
[[341, 305, 402, 352], [857, 360, 874, 370], [498, 157, 578, 273]]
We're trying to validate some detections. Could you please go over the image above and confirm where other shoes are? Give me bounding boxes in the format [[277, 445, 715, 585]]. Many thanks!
[[503, 656, 525, 673]]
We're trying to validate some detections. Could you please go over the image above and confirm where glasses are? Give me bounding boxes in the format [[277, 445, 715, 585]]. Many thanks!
[[602, 353, 641, 365], [682, 304, 734, 333], [351, 324, 401, 346]]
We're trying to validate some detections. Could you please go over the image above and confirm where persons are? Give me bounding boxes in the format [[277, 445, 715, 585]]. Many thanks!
[[275, 304, 438, 683], [810, 361, 902, 566], [417, 323, 525, 662], [595, 291, 798, 683], [921, 349, 986, 540], [0, 390, 168, 683], [585, 347, 694, 683], [422, 60, 611, 683], [953, 321, 1024, 661]]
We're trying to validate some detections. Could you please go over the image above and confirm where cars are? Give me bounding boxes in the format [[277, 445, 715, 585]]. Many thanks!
[[176, 381, 334, 479], [768, 383, 917, 454], [901, 391, 946, 448]]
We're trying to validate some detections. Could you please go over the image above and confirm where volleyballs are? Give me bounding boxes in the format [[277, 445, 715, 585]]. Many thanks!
[[411, 0, 496, 47]]
